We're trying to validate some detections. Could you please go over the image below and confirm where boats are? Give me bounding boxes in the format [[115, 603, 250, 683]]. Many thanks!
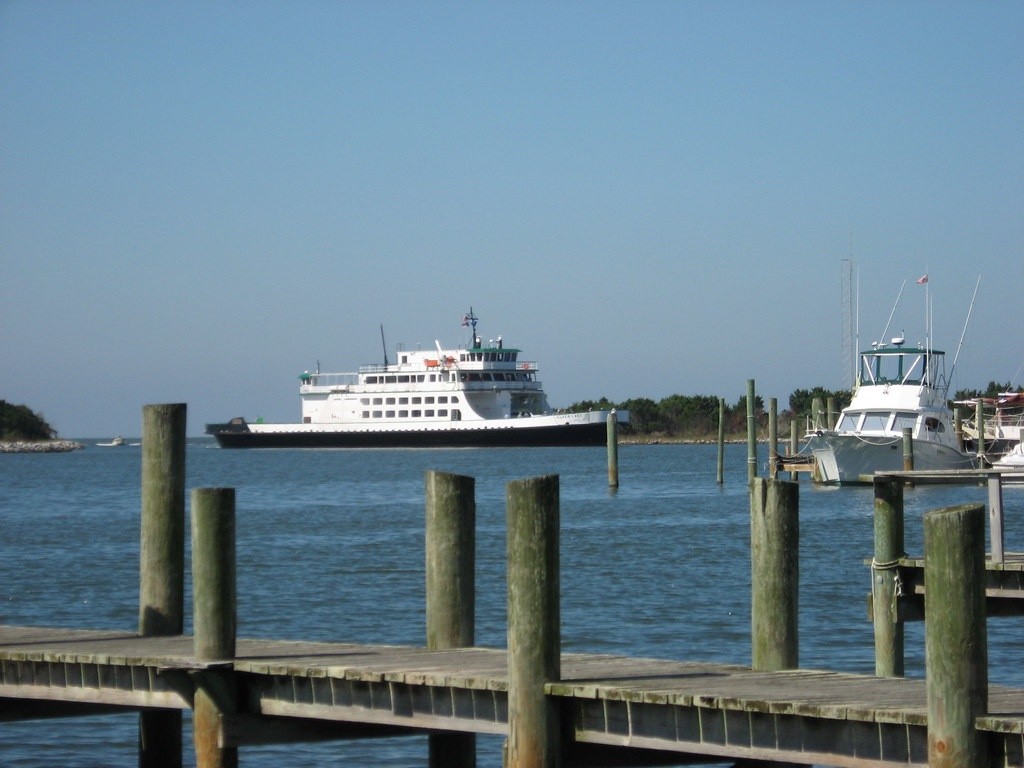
[[114, 436, 124, 445], [989, 440, 1024, 482], [803, 264, 981, 486], [201, 308, 631, 449]]
